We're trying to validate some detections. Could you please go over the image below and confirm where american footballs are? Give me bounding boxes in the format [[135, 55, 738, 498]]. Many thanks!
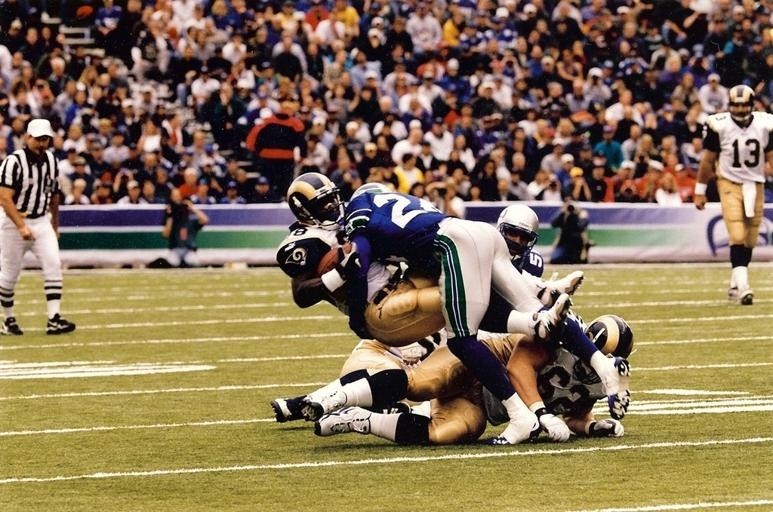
[[317, 244, 353, 277]]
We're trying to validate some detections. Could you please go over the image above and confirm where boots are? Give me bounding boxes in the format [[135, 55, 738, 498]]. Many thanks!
[[727, 267, 738, 298], [589, 350, 630, 421], [732, 267, 754, 304], [487, 392, 539, 446]]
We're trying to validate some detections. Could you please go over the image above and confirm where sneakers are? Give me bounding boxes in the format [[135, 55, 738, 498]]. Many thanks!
[[530, 294, 572, 342], [271, 395, 323, 423], [370, 402, 413, 417], [314, 406, 372, 436], [536, 270, 583, 307], [45, 313, 75, 334], [1, 317, 23, 335]]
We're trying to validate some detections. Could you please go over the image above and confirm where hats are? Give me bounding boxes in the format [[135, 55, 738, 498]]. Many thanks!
[[27, 119, 55, 139]]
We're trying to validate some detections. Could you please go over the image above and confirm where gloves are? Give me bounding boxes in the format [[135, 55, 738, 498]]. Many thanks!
[[582, 419, 623, 438], [539, 414, 570, 442], [336, 242, 361, 280], [348, 320, 373, 343]]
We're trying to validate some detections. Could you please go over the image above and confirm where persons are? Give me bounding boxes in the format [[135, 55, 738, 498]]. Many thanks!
[[1, 0, 772, 337], [270, 172, 634, 447]]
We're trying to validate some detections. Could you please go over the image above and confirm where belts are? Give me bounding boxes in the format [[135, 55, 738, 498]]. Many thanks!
[[22, 212, 45, 218], [373, 276, 395, 304]]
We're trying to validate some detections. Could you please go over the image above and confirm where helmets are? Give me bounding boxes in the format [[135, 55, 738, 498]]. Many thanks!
[[349, 183, 390, 201], [586, 314, 632, 359], [286, 173, 347, 226], [496, 203, 540, 256], [728, 86, 755, 123]]
